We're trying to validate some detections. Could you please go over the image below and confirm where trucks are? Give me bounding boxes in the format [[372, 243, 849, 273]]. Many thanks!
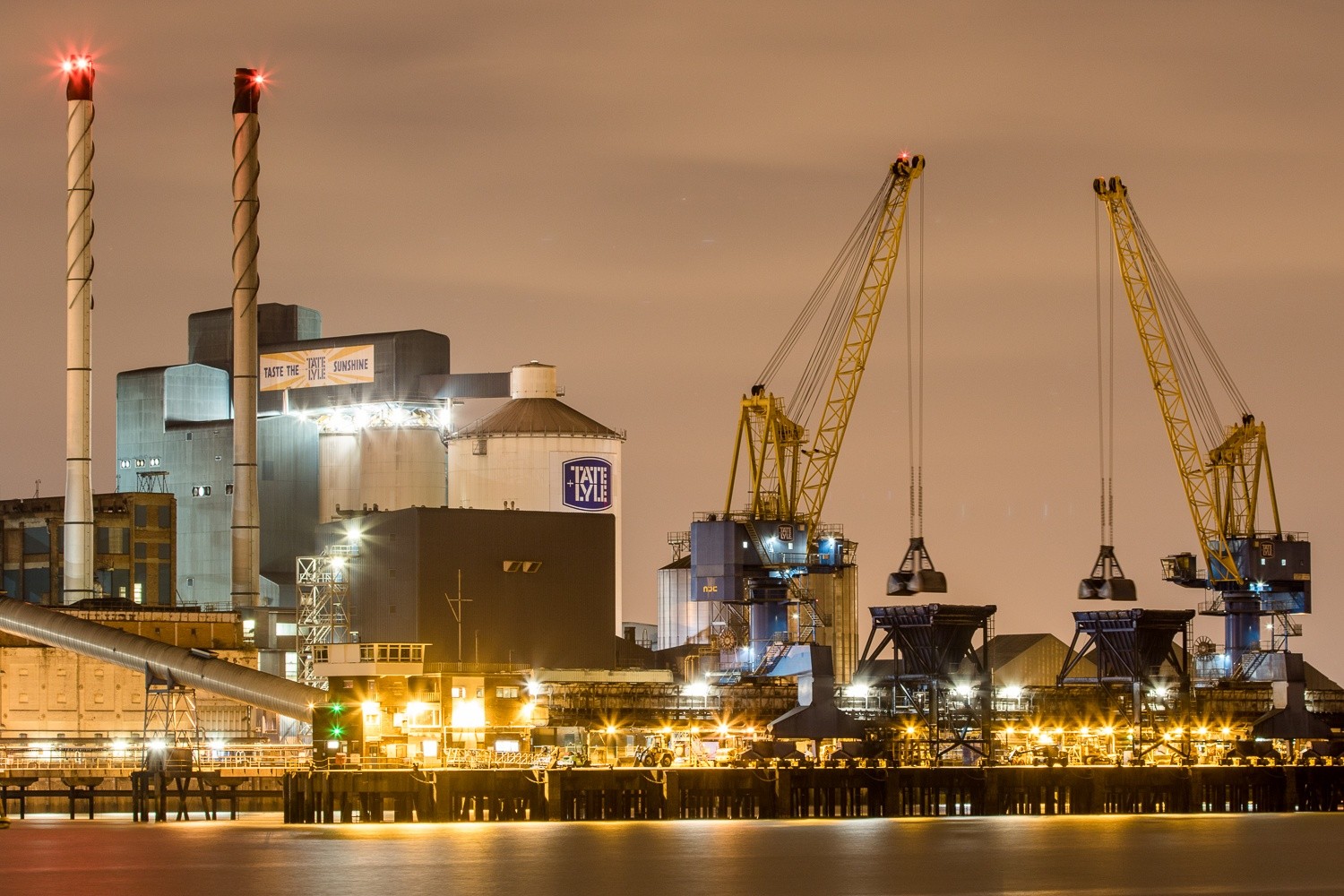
[[715, 735, 797, 769]]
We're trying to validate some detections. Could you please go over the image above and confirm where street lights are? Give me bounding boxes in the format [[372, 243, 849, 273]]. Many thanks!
[[1006, 728, 1013, 750], [604, 724, 614, 764], [1031, 729, 1038, 740], [229, 68, 263, 615], [1080, 728, 1087, 748], [1106, 727, 1111, 754], [65, 53, 98, 611], [1056, 728, 1063, 751], [689, 722, 698, 765], [1222, 728, 1228, 757]]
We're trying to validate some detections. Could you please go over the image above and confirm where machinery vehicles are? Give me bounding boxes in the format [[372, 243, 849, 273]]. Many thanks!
[[615, 736, 675, 768]]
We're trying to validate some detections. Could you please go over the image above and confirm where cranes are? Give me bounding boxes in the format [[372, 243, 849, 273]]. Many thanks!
[[688, 152, 948, 684], [1091, 174, 1314, 684]]
